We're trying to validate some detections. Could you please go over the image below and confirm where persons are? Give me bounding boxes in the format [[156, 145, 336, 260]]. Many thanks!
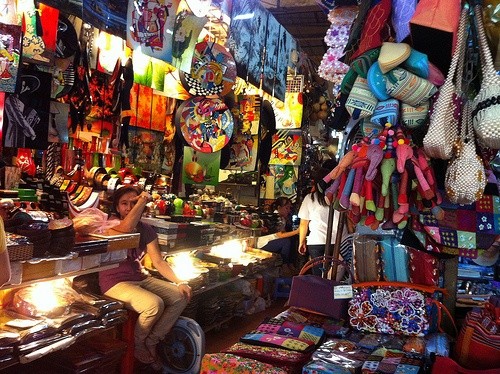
[[298, 159, 338, 275], [264, 197, 299, 275], [98, 187, 192, 369]]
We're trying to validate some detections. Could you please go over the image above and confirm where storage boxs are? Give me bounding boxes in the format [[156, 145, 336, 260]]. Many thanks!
[[201, 223, 210, 229], [200, 228, 216, 234], [88, 227, 141, 253], [110, 249, 127, 263], [141, 217, 178, 240], [72, 235, 108, 258], [55, 256, 83, 276], [157, 239, 176, 251], [81, 254, 101, 272], [22, 261, 56, 282], [98, 251, 111, 265], [190, 222, 202, 240], [177, 223, 193, 239], [201, 234, 215, 244]]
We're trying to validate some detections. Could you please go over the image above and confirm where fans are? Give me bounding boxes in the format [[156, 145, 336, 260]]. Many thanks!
[[156, 313, 206, 374]]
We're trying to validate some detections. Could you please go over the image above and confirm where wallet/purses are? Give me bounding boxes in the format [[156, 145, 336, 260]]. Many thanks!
[[199, 308, 350, 374], [340, 42, 444, 130]]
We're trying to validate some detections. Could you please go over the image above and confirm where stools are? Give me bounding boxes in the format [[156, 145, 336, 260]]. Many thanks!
[[121, 317, 138, 374], [272, 276, 293, 302]]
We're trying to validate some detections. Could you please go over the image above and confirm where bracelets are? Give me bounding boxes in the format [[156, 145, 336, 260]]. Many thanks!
[[177, 282, 188, 287], [140, 195, 147, 200]]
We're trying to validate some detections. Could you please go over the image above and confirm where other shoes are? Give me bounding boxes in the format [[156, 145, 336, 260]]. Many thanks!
[[282, 263, 296, 274], [133, 349, 161, 371]]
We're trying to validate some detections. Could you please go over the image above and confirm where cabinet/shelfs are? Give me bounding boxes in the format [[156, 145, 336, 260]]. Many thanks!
[[185, 259, 279, 333]]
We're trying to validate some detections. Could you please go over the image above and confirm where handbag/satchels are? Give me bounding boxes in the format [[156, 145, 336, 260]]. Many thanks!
[[409, 0, 500, 251], [285, 255, 500, 374]]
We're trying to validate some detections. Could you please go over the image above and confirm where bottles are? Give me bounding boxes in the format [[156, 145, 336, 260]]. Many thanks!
[[62, 134, 122, 170]]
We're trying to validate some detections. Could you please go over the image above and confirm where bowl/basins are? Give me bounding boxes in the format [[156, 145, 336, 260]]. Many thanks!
[[3, 211, 76, 259]]
